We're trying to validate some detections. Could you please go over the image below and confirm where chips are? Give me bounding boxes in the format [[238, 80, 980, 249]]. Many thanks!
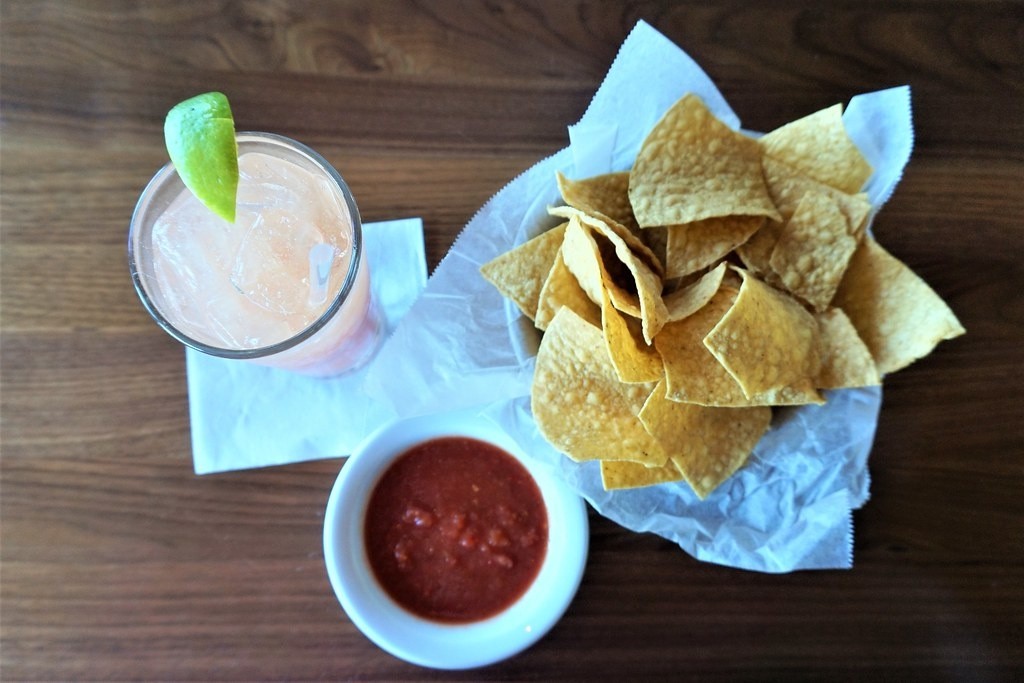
[[478, 95, 967, 502]]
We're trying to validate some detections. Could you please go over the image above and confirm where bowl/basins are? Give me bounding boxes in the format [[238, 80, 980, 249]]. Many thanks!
[[323, 406, 589, 670]]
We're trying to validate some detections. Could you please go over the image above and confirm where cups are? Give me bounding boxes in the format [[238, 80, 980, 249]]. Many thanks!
[[129, 134, 386, 378]]
